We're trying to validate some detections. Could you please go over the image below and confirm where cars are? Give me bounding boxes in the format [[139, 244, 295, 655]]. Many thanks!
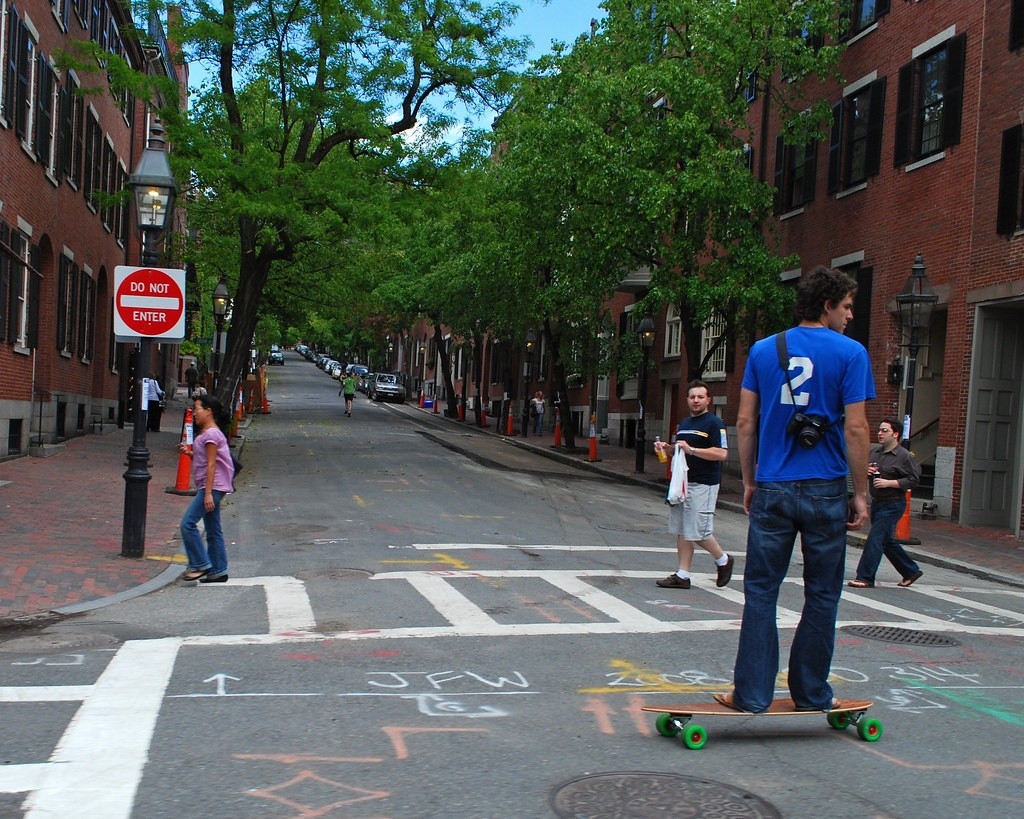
[[294, 344, 372, 392]]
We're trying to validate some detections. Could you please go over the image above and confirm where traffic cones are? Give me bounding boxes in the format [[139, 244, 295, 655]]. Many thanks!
[[890, 488, 922, 546]]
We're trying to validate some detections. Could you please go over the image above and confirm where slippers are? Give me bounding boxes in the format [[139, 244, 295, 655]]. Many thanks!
[[713, 693, 752, 713], [796, 697, 841, 710], [898, 570, 923, 587], [848, 579, 874, 588]]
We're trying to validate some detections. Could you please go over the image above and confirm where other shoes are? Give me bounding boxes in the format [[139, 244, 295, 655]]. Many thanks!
[[717, 554, 734, 586], [188, 397, 190, 398], [656, 574, 690, 589], [344, 409, 348, 414], [184, 569, 209, 580], [199, 573, 228, 583], [348, 411, 351, 417]]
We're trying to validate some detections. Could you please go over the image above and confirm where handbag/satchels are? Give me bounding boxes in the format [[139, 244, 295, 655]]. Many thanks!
[[667, 443, 689, 504], [157, 392, 164, 401]]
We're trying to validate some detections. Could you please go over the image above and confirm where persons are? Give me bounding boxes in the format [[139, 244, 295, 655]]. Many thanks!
[[654, 379, 734, 590], [146, 372, 165, 433], [847, 418, 923, 589], [179, 395, 234, 582], [530, 391, 546, 436], [338, 370, 361, 417], [714, 265, 876, 714], [185, 362, 208, 401]]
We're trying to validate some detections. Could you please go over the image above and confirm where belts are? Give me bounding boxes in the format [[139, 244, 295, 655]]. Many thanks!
[[872, 496, 901, 501]]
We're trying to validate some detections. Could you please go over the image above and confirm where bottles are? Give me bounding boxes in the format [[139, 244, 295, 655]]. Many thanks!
[[655, 436, 668, 463], [872, 463, 881, 481]]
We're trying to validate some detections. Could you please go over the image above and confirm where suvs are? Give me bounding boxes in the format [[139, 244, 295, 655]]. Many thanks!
[[367, 372, 407, 403], [268, 351, 284, 366]]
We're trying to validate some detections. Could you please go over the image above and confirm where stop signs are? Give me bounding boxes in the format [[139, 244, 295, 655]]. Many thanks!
[[112, 265, 186, 337]]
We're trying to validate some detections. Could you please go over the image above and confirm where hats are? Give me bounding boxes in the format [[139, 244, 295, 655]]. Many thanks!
[[191, 362, 194, 365]]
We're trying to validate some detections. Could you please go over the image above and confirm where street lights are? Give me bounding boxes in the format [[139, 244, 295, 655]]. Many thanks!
[[212, 274, 231, 395], [520, 328, 537, 438], [461, 338, 472, 419], [388, 342, 393, 372], [896, 250, 939, 450], [635, 307, 657, 473], [122, 116, 176, 560], [417, 343, 426, 404]]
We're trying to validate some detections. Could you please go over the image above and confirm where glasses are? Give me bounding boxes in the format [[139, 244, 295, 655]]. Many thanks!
[[878, 428, 892, 432]]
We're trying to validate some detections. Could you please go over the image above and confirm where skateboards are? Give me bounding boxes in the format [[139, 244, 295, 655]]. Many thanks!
[[640, 698, 882, 750]]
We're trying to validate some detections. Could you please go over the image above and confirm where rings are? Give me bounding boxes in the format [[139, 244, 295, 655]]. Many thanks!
[[860, 523, 864, 527]]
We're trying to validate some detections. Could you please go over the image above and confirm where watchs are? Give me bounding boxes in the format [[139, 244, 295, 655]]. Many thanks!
[[689, 447, 695, 456]]
